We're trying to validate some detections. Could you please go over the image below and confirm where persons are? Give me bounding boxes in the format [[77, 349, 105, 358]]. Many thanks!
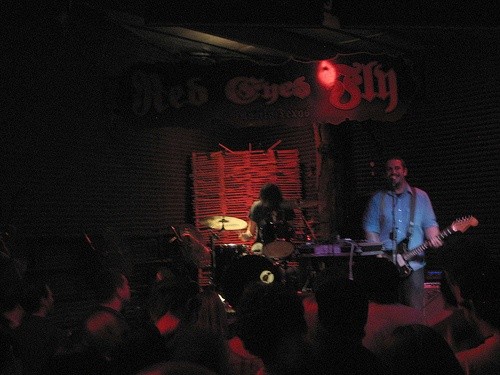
[[238, 182, 296, 254], [361, 157, 443, 314], [0, 255, 500, 375]]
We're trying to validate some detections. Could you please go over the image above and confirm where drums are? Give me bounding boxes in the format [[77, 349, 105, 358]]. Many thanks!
[[212, 243, 246, 275], [258, 220, 296, 258], [226, 252, 282, 293]]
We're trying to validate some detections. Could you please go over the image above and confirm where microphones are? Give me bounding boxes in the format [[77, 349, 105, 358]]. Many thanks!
[[209, 230, 220, 240], [390, 175, 397, 186]]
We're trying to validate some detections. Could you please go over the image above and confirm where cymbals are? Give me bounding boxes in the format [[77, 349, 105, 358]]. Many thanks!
[[278, 200, 319, 208], [296, 220, 329, 229], [198, 216, 247, 231]]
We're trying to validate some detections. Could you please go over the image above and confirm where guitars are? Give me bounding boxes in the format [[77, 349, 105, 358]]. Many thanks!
[[384, 215, 479, 278]]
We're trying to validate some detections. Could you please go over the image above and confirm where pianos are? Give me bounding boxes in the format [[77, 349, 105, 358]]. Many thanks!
[[291, 244, 382, 289]]
